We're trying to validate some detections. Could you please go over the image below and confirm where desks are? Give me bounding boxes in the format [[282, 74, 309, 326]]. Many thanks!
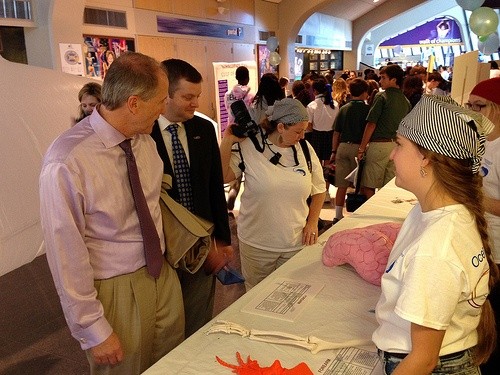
[[139, 176, 418, 375]]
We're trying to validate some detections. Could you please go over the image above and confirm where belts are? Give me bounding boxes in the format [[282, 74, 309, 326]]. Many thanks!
[[377, 349, 466, 359], [340, 141, 361, 144], [369, 138, 396, 142]]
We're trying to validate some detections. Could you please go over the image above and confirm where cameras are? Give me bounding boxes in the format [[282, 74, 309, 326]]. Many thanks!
[[230, 100, 259, 138]]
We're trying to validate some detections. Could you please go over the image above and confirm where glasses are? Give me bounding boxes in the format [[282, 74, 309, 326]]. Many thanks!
[[464, 103, 496, 112]]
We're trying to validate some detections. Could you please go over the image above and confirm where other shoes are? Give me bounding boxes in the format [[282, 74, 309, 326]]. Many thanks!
[[324, 191, 331, 201], [332, 216, 344, 226]]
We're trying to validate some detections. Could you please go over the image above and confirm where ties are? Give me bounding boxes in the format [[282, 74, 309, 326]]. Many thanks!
[[165, 123, 196, 214], [119, 138, 163, 279]]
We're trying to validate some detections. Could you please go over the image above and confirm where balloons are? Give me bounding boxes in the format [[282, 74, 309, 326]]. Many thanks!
[[270, 51, 281, 66], [478, 33, 499, 55], [478, 36, 487, 42], [267, 37, 279, 51], [469, 7, 498, 36]]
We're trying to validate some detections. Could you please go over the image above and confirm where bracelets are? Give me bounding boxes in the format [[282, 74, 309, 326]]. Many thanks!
[[331, 150, 338, 154], [357, 148, 366, 153]]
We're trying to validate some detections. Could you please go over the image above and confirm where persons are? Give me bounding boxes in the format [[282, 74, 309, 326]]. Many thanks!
[[219, 60, 498, 297], [39, 52, 185, 375], [150, 58, 232, 339], [436, 20, 451, 40], [78, 82, 102, 118], [372, 94, 499, 375], [463, 77, 500, 374]]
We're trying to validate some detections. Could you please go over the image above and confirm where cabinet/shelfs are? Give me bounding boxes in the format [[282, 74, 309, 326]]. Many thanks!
[[303, 50, 343, 75]]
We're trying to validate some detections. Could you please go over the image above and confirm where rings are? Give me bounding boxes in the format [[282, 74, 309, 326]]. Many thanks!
[[313, 233, 316, 235]]
